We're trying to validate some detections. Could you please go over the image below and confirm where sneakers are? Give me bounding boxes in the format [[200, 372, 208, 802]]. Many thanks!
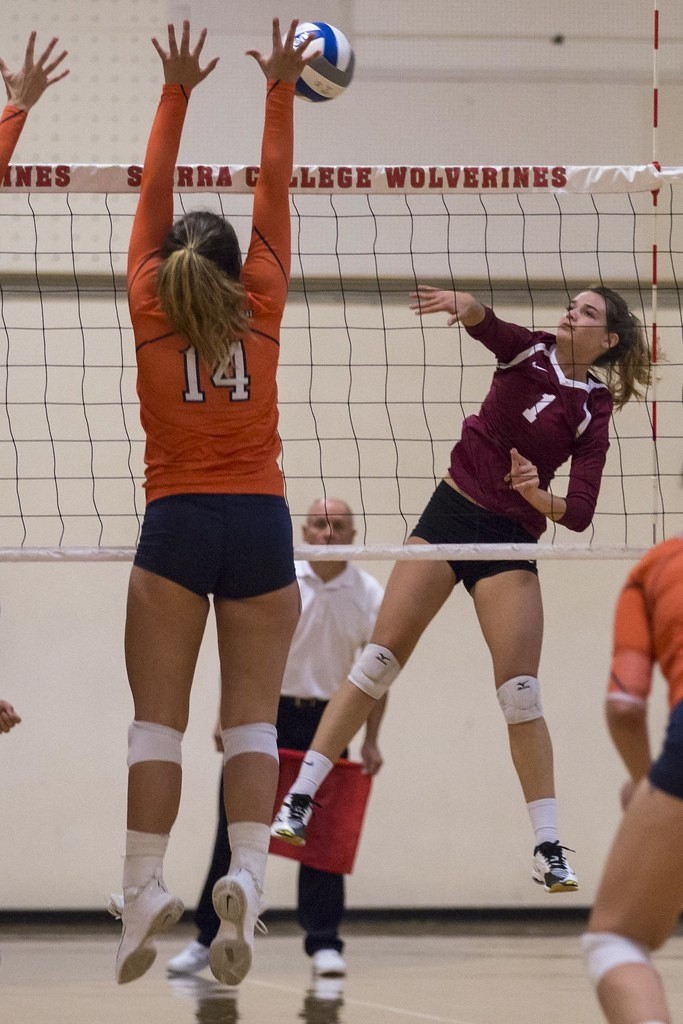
[[106, 877, 187, 985], [531, 840, 579, 893], [269, 791, 320, 844], [165, 937, 210, 979], [310, 946, 350, 978], [212, 872, 270, 988]]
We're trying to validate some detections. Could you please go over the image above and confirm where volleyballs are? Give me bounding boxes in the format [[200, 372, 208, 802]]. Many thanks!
[[280, 21, 356, 103]]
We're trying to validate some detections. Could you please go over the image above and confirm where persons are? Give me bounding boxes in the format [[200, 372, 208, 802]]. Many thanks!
[[269, 284, 653, 891], [165, 498, 384, 976], [115, 17, 323, 985], [582, 535, 683, 1023], [0, 29, 73, 184]]
